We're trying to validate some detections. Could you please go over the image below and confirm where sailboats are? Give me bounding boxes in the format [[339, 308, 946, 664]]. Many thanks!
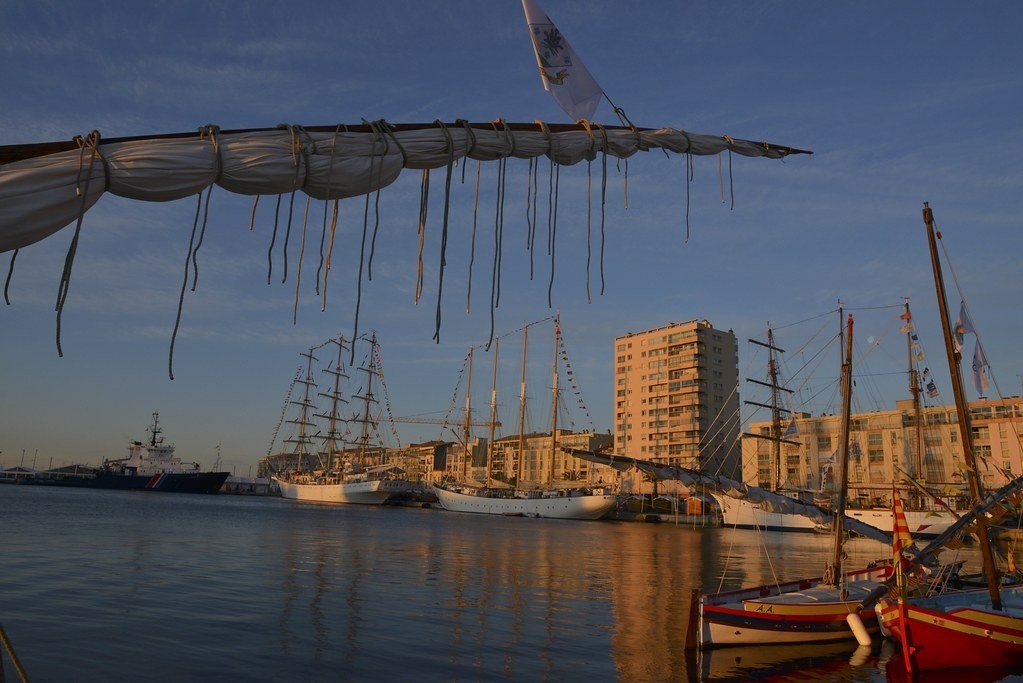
[[262, 327, 411, 506], [431, 307, 621, 521], [674, 297, 1023, 544], [873, 199, 1022, 676], [683, 305, 1023, 652]]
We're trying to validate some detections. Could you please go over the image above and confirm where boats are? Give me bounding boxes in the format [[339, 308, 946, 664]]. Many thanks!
[[93, 411, 231, 494]]
